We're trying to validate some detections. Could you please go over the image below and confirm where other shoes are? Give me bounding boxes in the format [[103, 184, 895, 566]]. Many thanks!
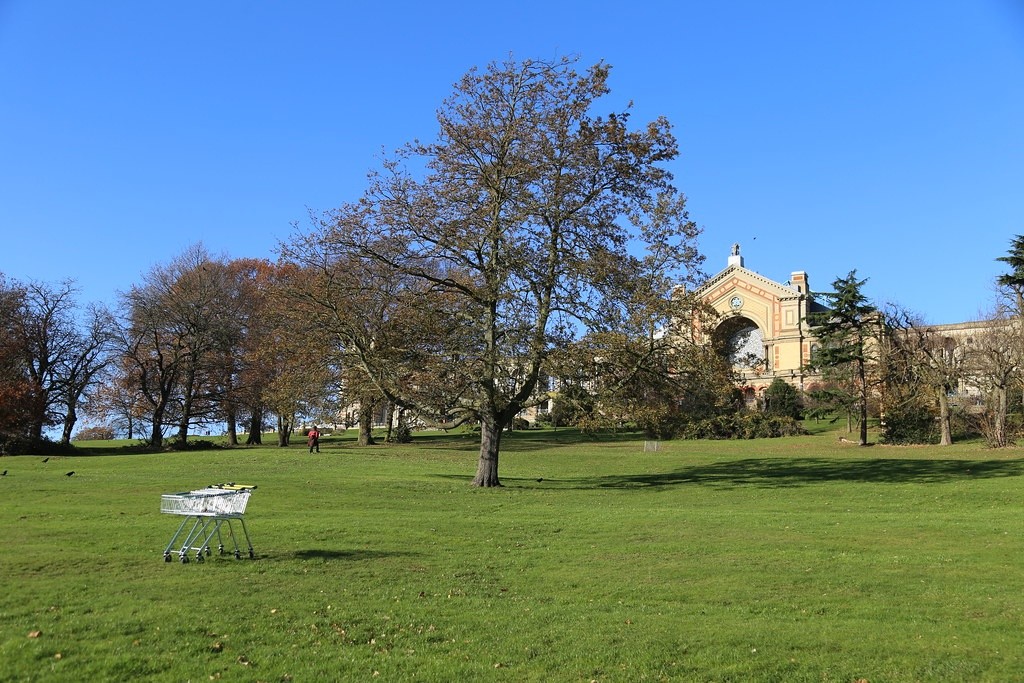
[[317, 451, 320, 452]]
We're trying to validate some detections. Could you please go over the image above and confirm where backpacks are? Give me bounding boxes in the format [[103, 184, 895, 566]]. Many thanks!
[[308, 435, 317, 446]]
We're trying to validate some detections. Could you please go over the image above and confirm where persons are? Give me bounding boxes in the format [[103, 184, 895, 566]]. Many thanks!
[[308, 425, 320, 454]]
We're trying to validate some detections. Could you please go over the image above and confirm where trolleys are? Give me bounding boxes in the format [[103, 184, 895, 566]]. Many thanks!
[[159, 482, 258, 564]]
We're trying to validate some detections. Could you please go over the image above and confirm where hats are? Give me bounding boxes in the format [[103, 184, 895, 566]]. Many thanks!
[[313, 426, 317, 428]]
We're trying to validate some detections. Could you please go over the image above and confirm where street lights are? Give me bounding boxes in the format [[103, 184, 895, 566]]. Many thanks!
[[798, 295, 805, 398]]
